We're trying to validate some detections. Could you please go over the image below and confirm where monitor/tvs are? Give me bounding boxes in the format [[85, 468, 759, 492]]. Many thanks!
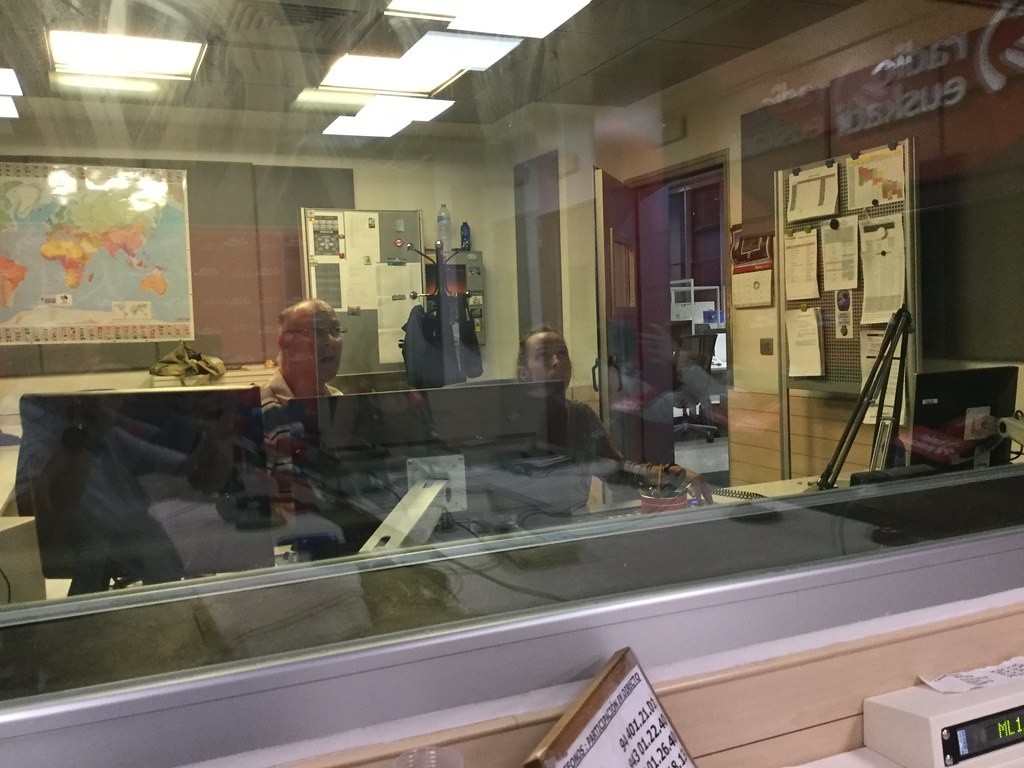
[[287, 378, 568, 563], [22, 384, 276, 581]]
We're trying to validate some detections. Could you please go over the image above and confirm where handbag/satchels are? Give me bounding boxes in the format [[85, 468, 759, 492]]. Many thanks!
[[148, 340, 228, 387], [398, 304, 465, 387], [458, 302, 484, 378]]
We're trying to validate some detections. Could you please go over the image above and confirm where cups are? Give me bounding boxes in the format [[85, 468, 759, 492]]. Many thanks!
[[641, 489, 687, 513]]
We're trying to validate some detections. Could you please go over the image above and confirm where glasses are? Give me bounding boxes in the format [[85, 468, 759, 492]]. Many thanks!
[[286, 325, 349, 340]]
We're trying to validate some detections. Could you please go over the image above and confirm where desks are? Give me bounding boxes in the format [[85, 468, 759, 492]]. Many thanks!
[[151, 369, 277, 389], [0, 424, 26, 517], [587, 468, 882, 506]]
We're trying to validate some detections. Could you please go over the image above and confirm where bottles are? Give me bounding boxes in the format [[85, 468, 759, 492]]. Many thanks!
[[437, 203, 451, 250], [461, 220, 471, 251]]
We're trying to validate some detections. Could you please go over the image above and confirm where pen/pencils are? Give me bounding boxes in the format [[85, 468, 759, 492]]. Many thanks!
[[637, 463, 692, 509]]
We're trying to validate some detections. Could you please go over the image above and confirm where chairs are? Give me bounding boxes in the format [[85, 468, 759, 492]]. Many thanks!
[[673, 334, 718, 443]]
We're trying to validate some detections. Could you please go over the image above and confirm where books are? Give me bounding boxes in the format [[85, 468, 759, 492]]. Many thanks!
[[728, 222, 775, 309]]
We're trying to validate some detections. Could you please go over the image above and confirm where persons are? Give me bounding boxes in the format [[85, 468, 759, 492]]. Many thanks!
[[640, 323, 762, 427], [517, 322, 710, 503], [260, 298, 348, 502]]
